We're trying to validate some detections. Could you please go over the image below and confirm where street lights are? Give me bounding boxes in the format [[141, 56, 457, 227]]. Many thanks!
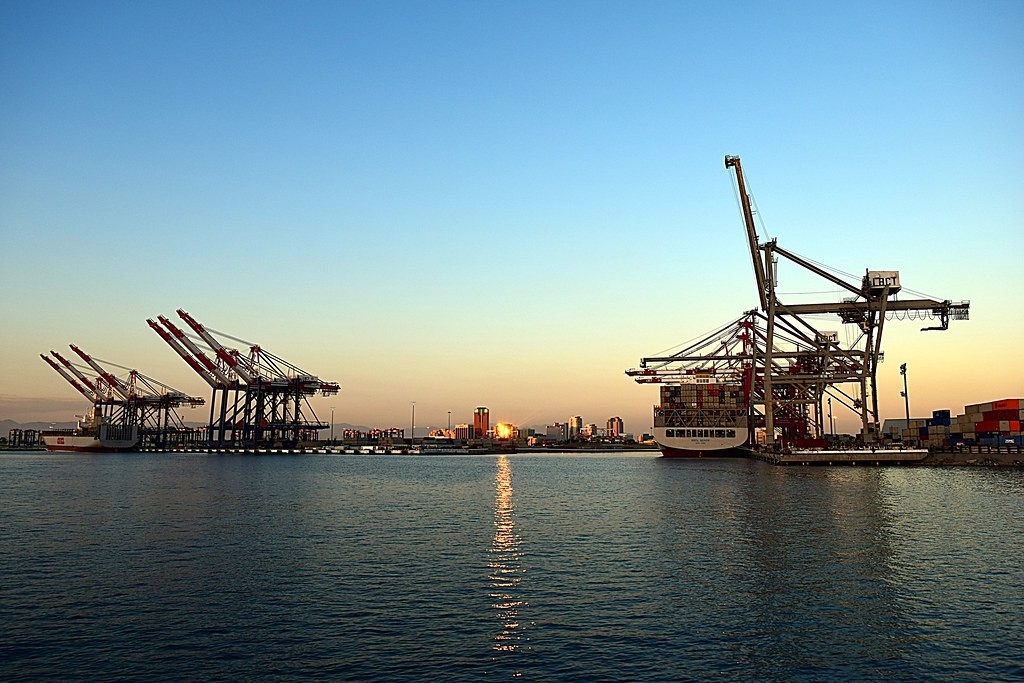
[[330, 408, 334, 440], [412, 402, 416, 444]]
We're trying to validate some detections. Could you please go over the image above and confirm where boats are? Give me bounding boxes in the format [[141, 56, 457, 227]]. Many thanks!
[[42, 424, 139, 453], [653, 404, 749, 458]]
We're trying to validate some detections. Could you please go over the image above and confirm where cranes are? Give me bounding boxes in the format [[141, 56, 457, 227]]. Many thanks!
[[157, 315, 295, 446], [49, 350, 180, 447], [39, 353, 147, 447], [70, 343, 207, 446], [626, 155, 971, 445], [177, 308, 341, 447], [146, 318, 283, 446]]
